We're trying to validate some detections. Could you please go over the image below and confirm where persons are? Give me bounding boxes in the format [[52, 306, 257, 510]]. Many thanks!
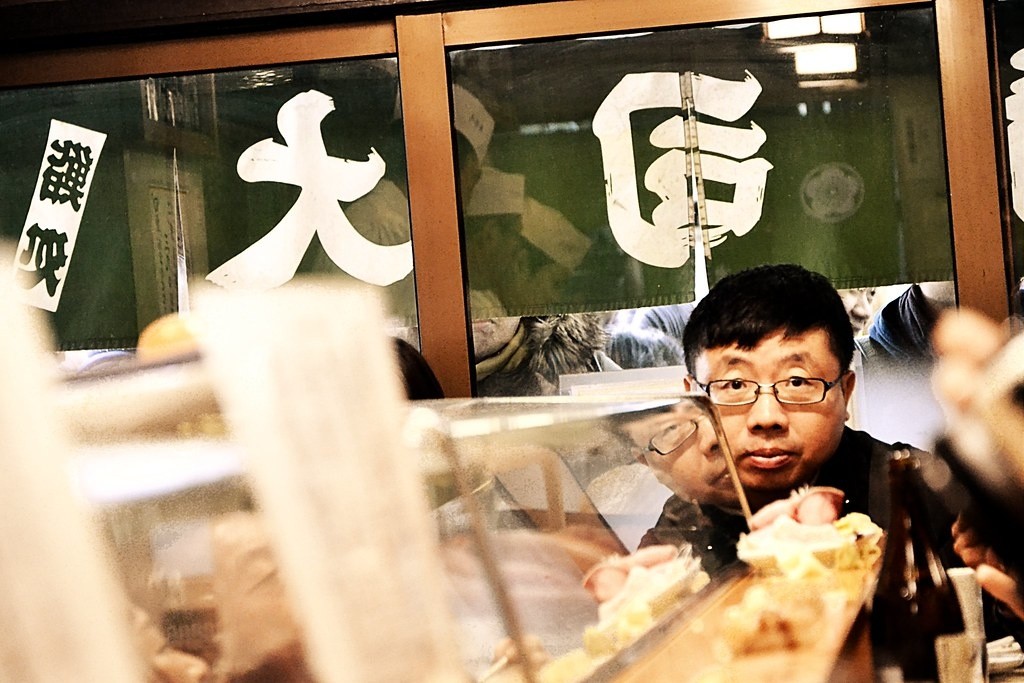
[[614, 404, 749, 572], [307, 81, 1024, 683]]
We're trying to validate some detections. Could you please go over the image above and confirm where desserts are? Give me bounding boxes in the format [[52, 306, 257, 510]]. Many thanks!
[[577, 543, 713, 662], [723, 484, 882, 655]]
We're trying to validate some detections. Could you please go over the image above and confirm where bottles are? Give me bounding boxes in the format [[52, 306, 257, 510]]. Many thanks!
[[868, 448, 976, 683]]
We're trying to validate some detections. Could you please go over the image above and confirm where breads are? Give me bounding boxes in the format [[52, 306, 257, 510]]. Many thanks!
[[134, 313, 202, 365]]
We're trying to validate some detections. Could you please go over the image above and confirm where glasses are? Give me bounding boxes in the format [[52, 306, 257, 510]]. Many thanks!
[[626, 412, 703, 465], [689, 369, 847, 406]]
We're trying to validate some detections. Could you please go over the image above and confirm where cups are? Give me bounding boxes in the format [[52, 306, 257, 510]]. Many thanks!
[[948, 569, 988, 683]]
